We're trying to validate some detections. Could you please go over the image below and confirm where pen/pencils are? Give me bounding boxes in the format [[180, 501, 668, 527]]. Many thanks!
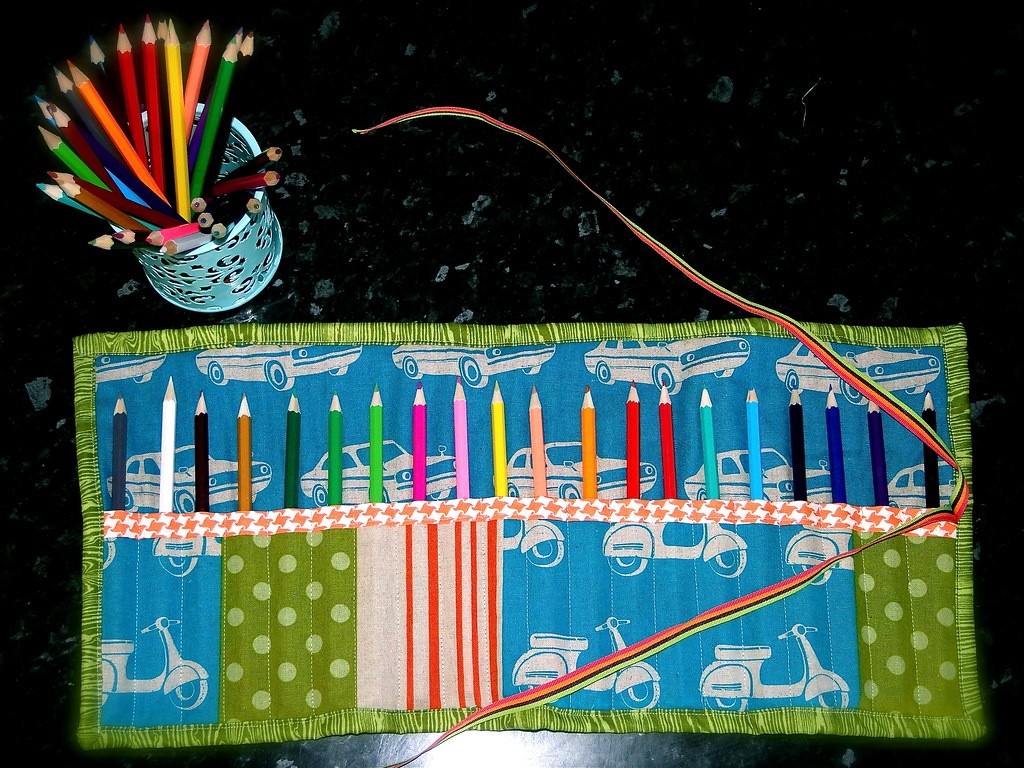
[[284, 388, 302, 509], [922, 390, 941, 508], [745, 380, 764, 500], [411, 380, 427, 502], [626, 380, 641, 500], [328, 390, 344, 506], [528, 386, 548, 497], [34, 11, 283, 261], [111, 390, 128, 511], [789, 385, 807, 501], [825, 384, 848, 503], [367, 383, 384, 504], [867, 400, 889, 507], [452, 376, 470, 498], [158, 375, 178, 513], [236, 391, 252, 511], [489, 380, 509, 497], [193, 389, 210, 513], [699, 383, 721, 500], [658, 381, 677, 500], [580, 385, 598, 500]]
